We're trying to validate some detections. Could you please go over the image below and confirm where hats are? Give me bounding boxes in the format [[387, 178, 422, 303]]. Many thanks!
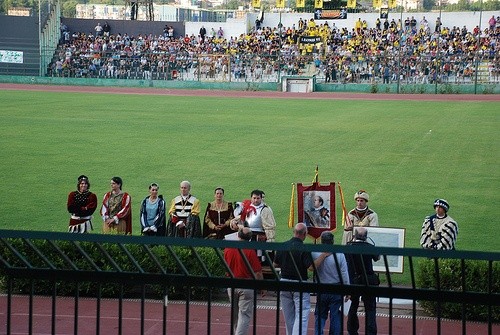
[[354, 189, 368, 202], [77, 175, 91, 190], [433, 198, 449, 212]]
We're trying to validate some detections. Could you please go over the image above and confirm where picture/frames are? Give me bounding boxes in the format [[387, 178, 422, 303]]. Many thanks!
[[352, 225, 405, 274]]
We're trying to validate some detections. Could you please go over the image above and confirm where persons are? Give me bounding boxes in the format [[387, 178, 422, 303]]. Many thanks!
[[381, 12, 387, 17], [140, 183, 166, 246], [230, 190, 276, 267], [167, 180, 202, 238], [224, 227, 267, 335], [273, 223, 333, 335], [46, 15, 500, 84], [343, 190, 379, 246], [66, 175, 98, 234], [202, 187, 234, 240], [344, 227, 381, 335], [303, 192, 330, 227], [311, 231, 351, 335], [100, 177, 132, 235], [420, 198, 458, 259]]
